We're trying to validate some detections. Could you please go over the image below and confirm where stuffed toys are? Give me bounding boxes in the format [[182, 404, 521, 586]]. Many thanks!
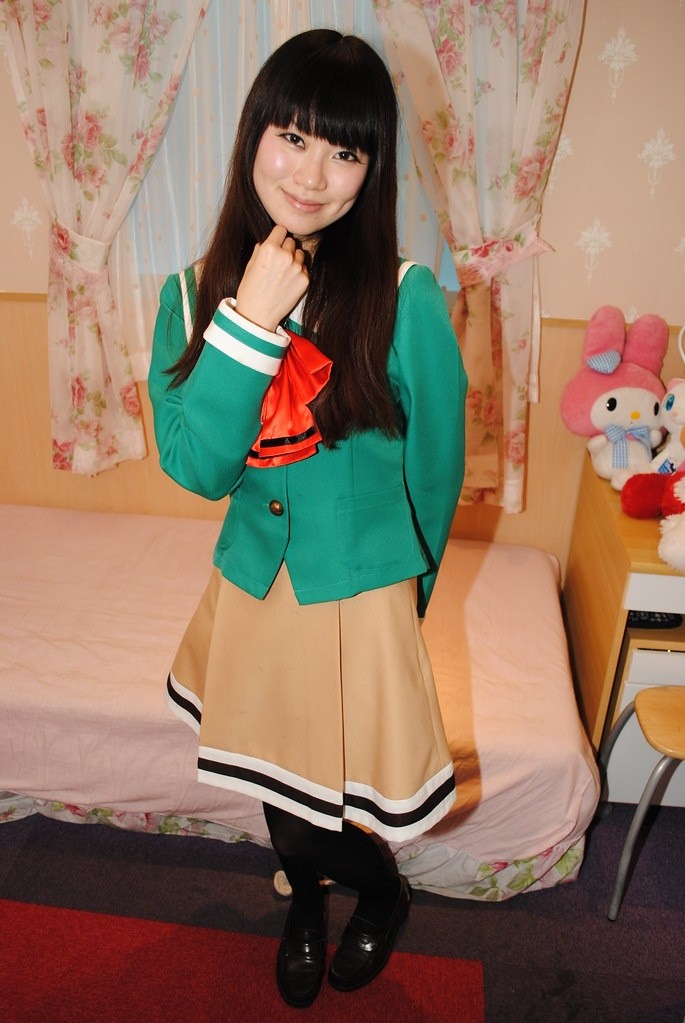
[[656, 477, 685, 575], [610, 378, 685, 491], [561, 304, 669, 480], [620, 468, 685, 519]]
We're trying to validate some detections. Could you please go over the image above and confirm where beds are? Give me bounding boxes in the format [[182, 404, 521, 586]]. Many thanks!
[[0, 502, 603, 902]]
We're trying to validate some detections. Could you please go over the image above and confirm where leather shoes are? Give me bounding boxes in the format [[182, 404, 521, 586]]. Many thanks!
[[276, 891, 326, 1009], [328, 873, 412, 992]]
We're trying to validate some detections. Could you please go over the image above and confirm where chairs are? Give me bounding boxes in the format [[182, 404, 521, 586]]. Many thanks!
[[594, 685, 685, 922]]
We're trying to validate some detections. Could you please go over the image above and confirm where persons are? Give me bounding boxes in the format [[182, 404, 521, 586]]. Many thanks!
[[149, 28, 468, 1007]]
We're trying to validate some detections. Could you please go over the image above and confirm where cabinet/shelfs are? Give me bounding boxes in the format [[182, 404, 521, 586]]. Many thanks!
[[563, 447, 685, 817]]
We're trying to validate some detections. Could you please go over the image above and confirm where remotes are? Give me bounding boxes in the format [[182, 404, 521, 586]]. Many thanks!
[[628, 610, 682, 629]]
